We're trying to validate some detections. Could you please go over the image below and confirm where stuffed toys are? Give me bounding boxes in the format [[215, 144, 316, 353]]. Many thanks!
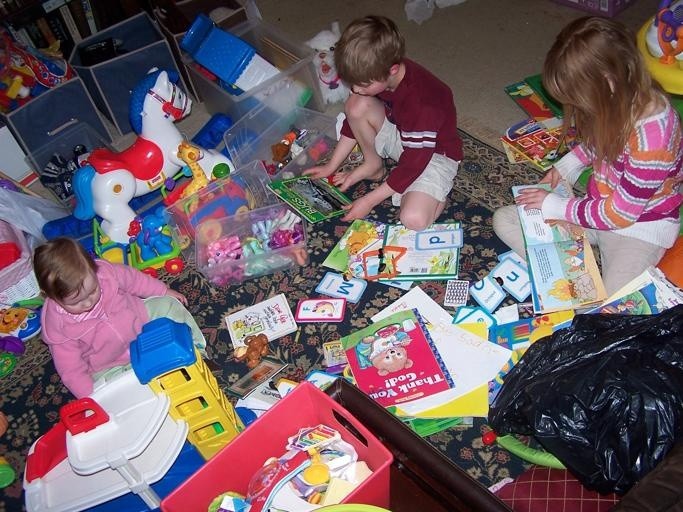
[[305, 22, 355, 105]]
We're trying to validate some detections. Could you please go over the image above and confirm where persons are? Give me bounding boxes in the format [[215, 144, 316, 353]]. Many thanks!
[[301, 15, 463, 232], [492, 15, 683, 314], [33, 237, 209, 399]]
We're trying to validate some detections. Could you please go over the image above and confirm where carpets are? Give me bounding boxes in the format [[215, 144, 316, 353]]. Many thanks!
[[0, 116, 604, 512]]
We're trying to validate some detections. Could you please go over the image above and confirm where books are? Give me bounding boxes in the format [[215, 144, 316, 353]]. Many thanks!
[[322, 218, 464, 292], [266, 173, 352, 224], [225, 293, 298, 348], [510, 180, 609, 315]]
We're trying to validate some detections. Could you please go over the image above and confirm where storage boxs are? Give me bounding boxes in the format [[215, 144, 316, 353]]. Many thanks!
[[158, 18, 343, 287]]
